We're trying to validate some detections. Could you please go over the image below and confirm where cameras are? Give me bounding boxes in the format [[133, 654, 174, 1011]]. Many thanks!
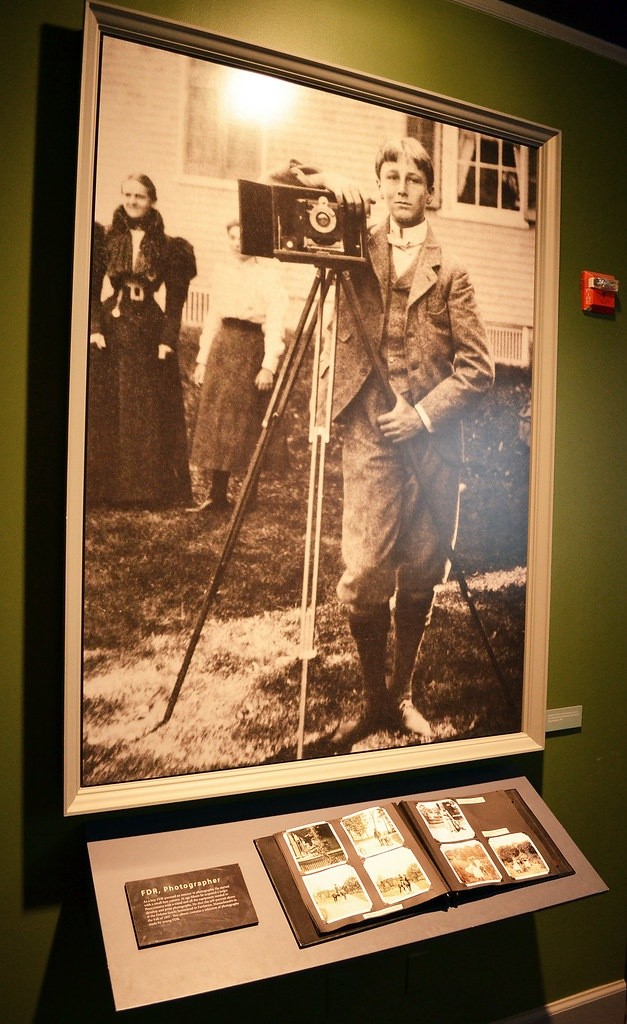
[[239, 179, 367, 269]]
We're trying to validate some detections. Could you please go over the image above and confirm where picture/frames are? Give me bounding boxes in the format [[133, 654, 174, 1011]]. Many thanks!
[[63, 0, 563, 818]]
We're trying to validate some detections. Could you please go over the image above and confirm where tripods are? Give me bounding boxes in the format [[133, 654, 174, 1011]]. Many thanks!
[[165, 261, 521, 759]]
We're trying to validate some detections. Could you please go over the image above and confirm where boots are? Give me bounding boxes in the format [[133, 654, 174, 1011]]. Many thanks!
[[333, 608, 392, 745], [389, 600, 436, 735]]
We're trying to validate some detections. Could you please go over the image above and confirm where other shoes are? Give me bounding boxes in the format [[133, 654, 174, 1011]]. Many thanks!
[[247, 485, 260, 511], [184, 486, 230, 513]]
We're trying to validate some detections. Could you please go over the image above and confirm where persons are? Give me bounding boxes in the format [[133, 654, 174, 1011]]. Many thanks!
[[230, 135, 495, 748], [280, 799, 533, 909], [194, 220, 283, 513], [96, 174, 193, 508]]
